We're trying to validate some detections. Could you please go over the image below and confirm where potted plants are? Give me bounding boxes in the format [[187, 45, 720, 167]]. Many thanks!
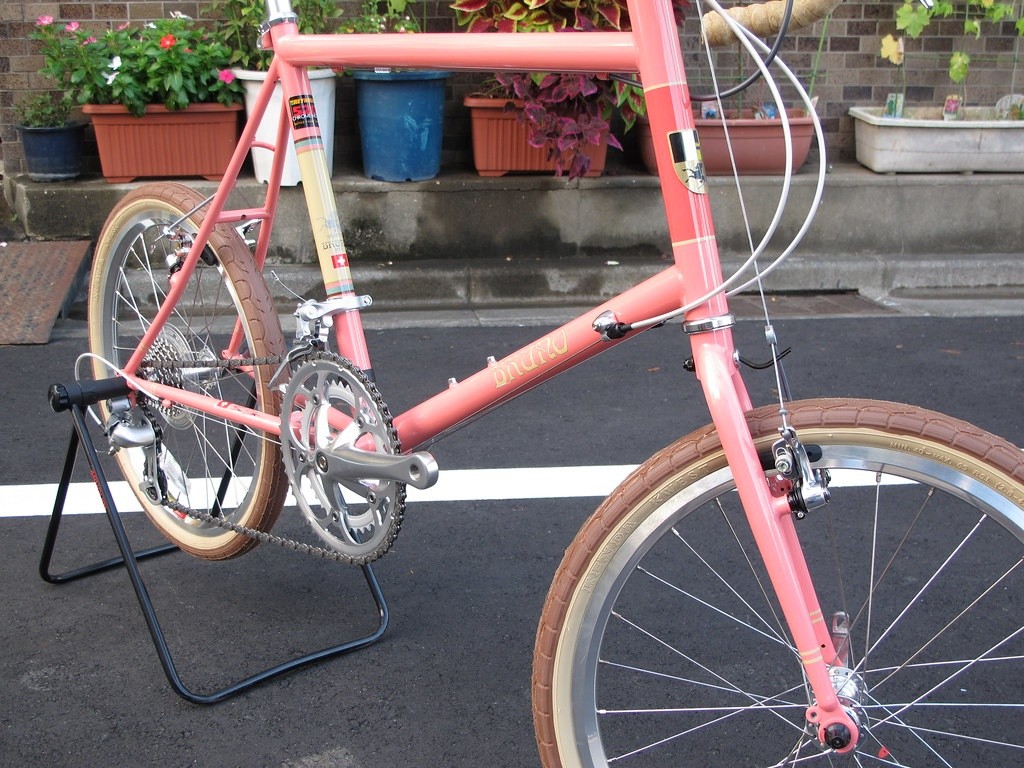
[[849, 0, 1024, 175], [636, 12, 830, 177], [7, 87, 90, 182], [25, 10, 244, 184], [200, 0, 344, 186], [332, 0, 457, 182], [449, 0, 695, 187]]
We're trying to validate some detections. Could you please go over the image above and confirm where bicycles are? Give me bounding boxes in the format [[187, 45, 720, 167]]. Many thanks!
[[73, 0, 1024, 767]]
